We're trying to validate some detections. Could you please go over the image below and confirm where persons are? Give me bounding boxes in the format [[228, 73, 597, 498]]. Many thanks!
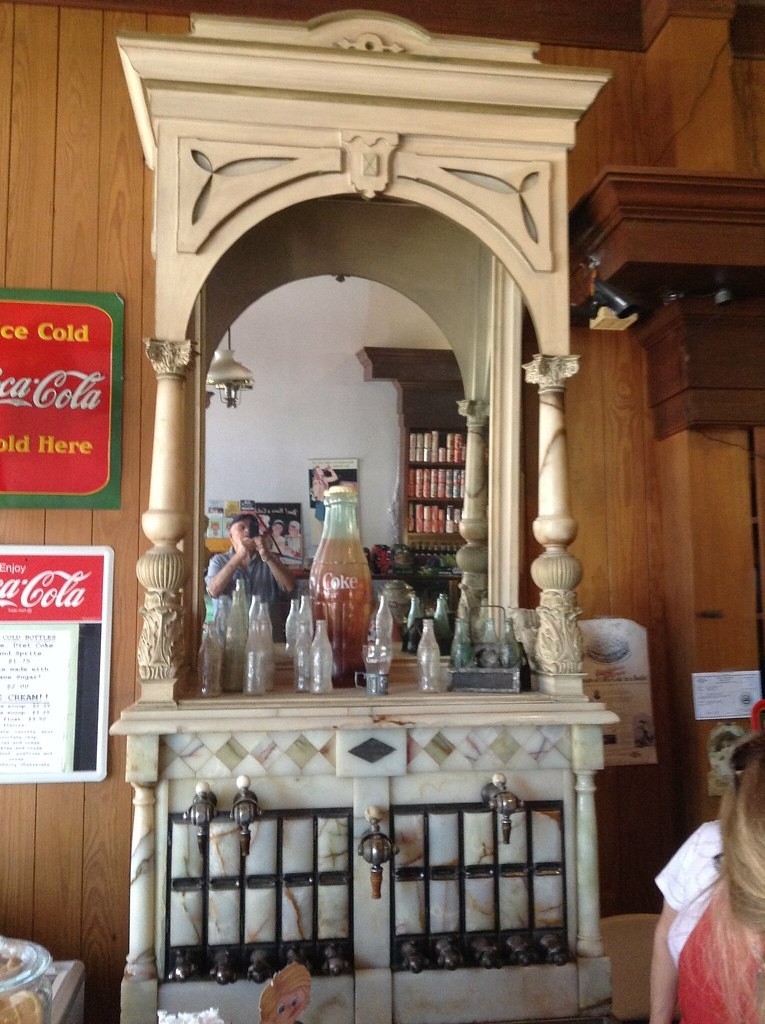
[[205, 515, 294, 647], [650, 732, 765, 1024]]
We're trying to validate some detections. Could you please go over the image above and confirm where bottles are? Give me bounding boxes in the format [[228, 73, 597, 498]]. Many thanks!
[[310, 620, 333, 695], [375, 595, 394, 646], [315, 485, 372, 688], [197, 621, 222, 697], [258, 601, 273, 642], [412, 544, 463, 570], [293, 621, 311, 692], [451, 619, 471, 668], [480, 618, 498, 668], [298, 594, 315, 645], [234, 578, 249, 621], [406, 596, 421, 651], [433, 599, 449, 641], [214, 595, 230, 651], [249, 593, 260, 630], [257, 622, 274, 691], [309, 489, 330, 629], [499, 618, 521, 668], [244, 621, 263, 696], [284, 599, 300, 657], [438, 593, 449, 613], [417, 618, 440, 694], [225, 591, 248, 690]]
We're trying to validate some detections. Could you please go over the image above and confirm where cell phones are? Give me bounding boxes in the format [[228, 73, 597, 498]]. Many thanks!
[[248, 523, 259, 539]]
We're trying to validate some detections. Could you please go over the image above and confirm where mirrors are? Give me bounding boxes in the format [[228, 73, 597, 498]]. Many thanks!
[[203, 272, 467, 652]]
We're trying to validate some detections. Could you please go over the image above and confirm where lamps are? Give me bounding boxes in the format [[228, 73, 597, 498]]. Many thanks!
[[205, 327, 256, 408]]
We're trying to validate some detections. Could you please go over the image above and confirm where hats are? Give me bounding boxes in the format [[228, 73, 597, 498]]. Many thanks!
[[231, 514, 259, 525]]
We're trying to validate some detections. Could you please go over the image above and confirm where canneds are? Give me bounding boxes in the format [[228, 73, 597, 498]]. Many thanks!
[[408, 430, 468, 534]]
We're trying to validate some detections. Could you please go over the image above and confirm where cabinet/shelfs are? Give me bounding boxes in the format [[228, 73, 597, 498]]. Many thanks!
[[407, 428, 467, 535]]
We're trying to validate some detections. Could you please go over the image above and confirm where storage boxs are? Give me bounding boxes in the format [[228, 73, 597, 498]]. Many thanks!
[[448, 604, 532, 694]]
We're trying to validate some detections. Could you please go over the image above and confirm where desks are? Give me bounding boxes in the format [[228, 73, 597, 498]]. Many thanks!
[[108, 674, 622, 1024]]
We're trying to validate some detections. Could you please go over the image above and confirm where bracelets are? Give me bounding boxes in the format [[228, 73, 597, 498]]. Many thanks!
[[261, 555, 270, 562]]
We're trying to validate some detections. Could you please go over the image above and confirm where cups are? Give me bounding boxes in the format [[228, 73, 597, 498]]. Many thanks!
[[361, 644, 392, 694]]
